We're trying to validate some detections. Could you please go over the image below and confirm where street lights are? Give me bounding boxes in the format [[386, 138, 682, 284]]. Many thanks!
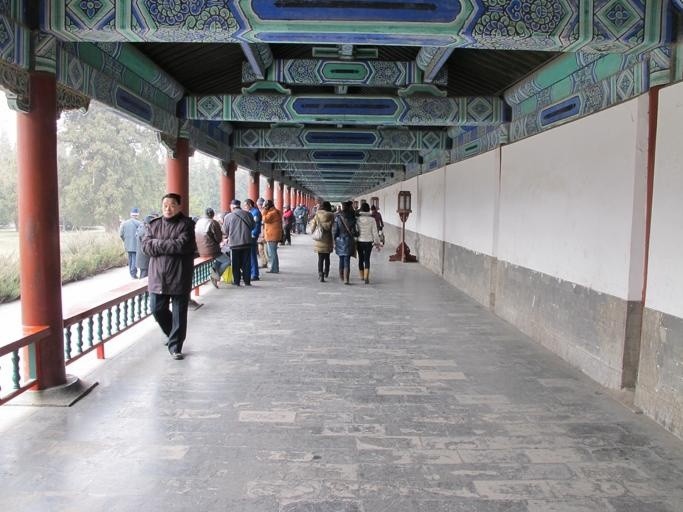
[[312, 215, 327, 241], [352, 239, 357, 258]]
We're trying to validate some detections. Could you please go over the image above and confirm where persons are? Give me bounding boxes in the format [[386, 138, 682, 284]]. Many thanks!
[[330, 200, 356, 285], [137, 194, 195, 360], [118, 207, 142, 279], [354, 203, 382, 284], [193, 207, 231, 288], [137, 196, 341, 309], [310, 201, 334, 282], [370, 205, 385, 252]]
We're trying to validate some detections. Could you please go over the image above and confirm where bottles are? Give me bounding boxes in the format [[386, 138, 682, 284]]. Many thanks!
[[130, 208, 139, 215], [230, 200, 240, 205]]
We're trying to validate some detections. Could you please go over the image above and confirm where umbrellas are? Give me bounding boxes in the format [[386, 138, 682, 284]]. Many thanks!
[[170, 352, 184, 360], [209, 276, 219, 288], [209, 266, 220, 281]]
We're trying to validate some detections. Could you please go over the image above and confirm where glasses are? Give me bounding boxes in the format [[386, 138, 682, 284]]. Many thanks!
[[369, 197, 380, 211]]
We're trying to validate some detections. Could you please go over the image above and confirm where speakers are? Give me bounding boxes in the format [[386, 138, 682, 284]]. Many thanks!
[[344, 271, 349, 284], [324, 272, 328, 278], [358, 270, 364, 280], [364, 269, 369, 283], [339, 269, 344, 279], [318, 272, 324, 282]]
